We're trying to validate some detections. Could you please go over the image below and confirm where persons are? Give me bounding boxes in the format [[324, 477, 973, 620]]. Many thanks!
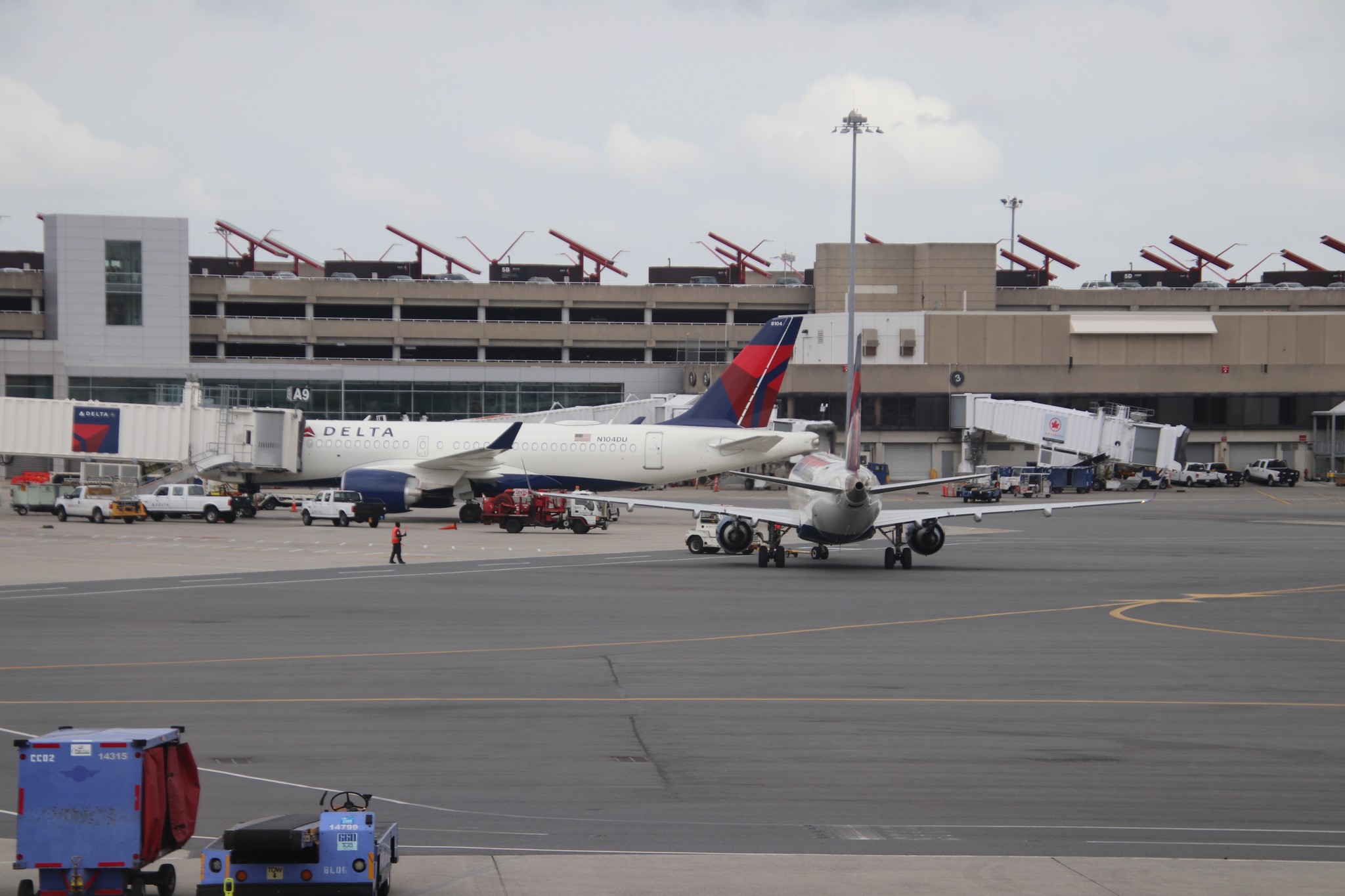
[[389, 522, 407, 564]]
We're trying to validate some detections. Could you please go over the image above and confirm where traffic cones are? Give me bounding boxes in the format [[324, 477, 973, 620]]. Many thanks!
[[289, 495, 299, 512], [481, 492, 488, 503], [438, 523, 457, 530]]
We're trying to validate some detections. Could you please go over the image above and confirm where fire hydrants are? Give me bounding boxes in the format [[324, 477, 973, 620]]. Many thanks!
[[1303, 468, 1309, 480]]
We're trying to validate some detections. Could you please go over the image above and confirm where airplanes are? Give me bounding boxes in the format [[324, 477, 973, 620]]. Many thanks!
[[528, 333, 1159, 572], [0, 314, 823, 508]]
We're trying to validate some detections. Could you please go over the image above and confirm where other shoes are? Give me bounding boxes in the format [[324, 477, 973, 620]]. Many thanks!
[[399, 561, 405, 564], [389, 561, 396, 563]]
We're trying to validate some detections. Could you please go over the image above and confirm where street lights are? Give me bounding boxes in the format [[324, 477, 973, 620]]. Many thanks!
[[832, 111, 884, 440], [997, 195, 1026, 277]]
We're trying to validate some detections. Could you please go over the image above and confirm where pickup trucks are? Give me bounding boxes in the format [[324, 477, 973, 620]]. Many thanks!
[[300, 489, 384, 527], [1169, 461, 1219, 487], [133, 483, 252, 523], [481, 485, 610, 534], [1244, 458, 1301, 486], [53, 485, 139, 524], [1202, 462, 1247, 487]]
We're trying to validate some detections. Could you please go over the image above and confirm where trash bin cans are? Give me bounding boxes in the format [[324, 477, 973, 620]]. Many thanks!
[[930, 469, 937, 480]]
